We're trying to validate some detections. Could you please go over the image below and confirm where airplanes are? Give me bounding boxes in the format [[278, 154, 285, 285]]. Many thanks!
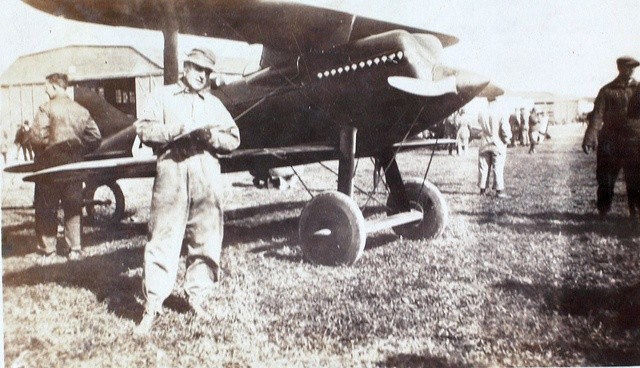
[[15, 10, 490, 257]]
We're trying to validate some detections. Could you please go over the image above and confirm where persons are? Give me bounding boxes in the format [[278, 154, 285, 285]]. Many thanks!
[[453, 94, 551, 200], [132, 47, 241, 345], [30, 73, 102, 254], [582, 57, 637, 228]]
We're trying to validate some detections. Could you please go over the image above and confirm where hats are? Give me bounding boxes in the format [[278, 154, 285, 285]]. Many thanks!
[[185, 47, 217, 72], [617, 57, 639, 67]]
